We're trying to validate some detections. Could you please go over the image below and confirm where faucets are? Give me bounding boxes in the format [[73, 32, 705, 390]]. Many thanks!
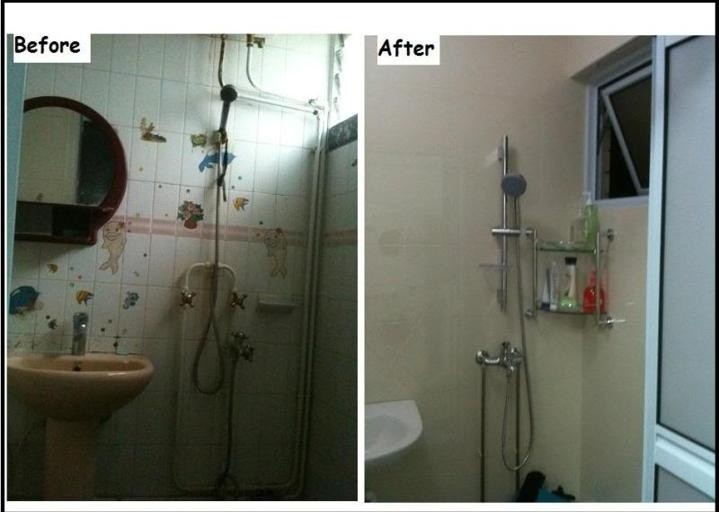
[[229, 331, 255, 364], [71, 310, 89, 355]]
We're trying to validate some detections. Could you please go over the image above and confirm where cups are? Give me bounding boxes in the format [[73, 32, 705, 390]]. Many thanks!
[[566, 219, 587, 249]]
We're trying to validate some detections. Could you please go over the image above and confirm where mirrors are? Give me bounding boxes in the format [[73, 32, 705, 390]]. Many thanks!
[[13, 96, 125, 245]]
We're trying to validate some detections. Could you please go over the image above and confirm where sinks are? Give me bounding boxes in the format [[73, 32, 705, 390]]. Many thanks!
[[8, 353, 154, 419], [364, 400, 423, 474]]
